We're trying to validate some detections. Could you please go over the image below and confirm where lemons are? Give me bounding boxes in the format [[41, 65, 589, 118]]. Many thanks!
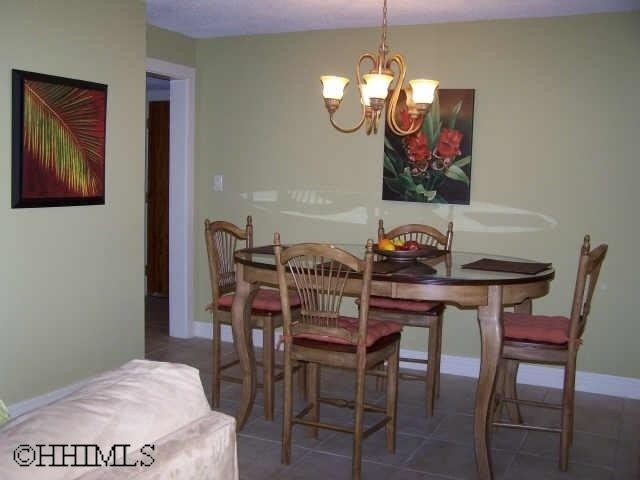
[[394, 240, 404, 246], [384, 244, 395, 251]]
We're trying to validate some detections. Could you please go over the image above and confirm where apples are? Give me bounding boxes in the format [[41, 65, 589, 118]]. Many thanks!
[[404, 241, 419, 250]]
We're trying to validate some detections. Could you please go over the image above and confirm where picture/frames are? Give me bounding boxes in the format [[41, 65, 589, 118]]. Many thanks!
[[11, 70, 107, 207]]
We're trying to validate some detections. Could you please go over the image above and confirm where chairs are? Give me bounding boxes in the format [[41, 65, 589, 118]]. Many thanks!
[[204, 214, 453, 480], [491, 234, 608, 471]]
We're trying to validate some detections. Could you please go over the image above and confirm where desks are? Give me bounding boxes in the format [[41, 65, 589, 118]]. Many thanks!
[[231, 244, 555, 480]]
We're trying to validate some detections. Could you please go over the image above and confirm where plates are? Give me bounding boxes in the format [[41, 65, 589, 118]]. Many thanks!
[[369, 260, 438, 276], [369, 243, 435, 261]]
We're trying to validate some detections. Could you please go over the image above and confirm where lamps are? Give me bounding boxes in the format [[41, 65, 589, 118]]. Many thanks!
[[319, 0, 439, 136]]
